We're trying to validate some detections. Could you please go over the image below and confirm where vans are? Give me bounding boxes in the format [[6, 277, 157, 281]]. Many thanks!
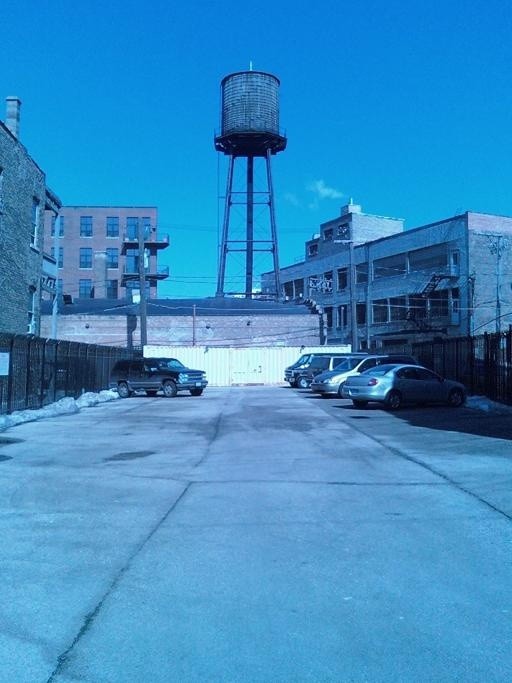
[[283, 352, 467, 409]]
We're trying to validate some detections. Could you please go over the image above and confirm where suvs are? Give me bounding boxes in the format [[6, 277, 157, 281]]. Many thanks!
[[110, 357, 207, 398]]
